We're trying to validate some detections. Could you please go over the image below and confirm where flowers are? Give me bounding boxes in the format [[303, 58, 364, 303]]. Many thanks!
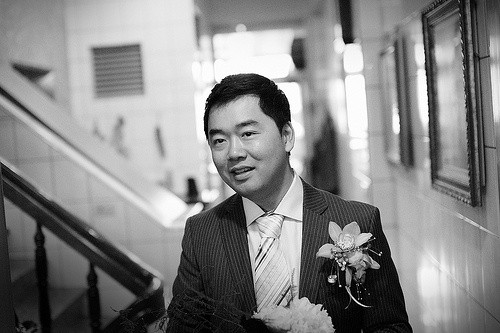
[[313, 219, 385, 311], [244, 297, 338, 333]]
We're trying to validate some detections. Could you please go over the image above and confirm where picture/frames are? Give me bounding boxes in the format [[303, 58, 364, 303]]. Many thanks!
[[418, 0, 487, 208], [377, 33, 417, 171]]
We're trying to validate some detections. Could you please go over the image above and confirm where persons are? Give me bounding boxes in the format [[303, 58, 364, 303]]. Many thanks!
[[167, 72, 416, 333]]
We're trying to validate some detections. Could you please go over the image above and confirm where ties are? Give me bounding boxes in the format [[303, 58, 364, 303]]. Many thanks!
[[253, 215, 293, 312]]
